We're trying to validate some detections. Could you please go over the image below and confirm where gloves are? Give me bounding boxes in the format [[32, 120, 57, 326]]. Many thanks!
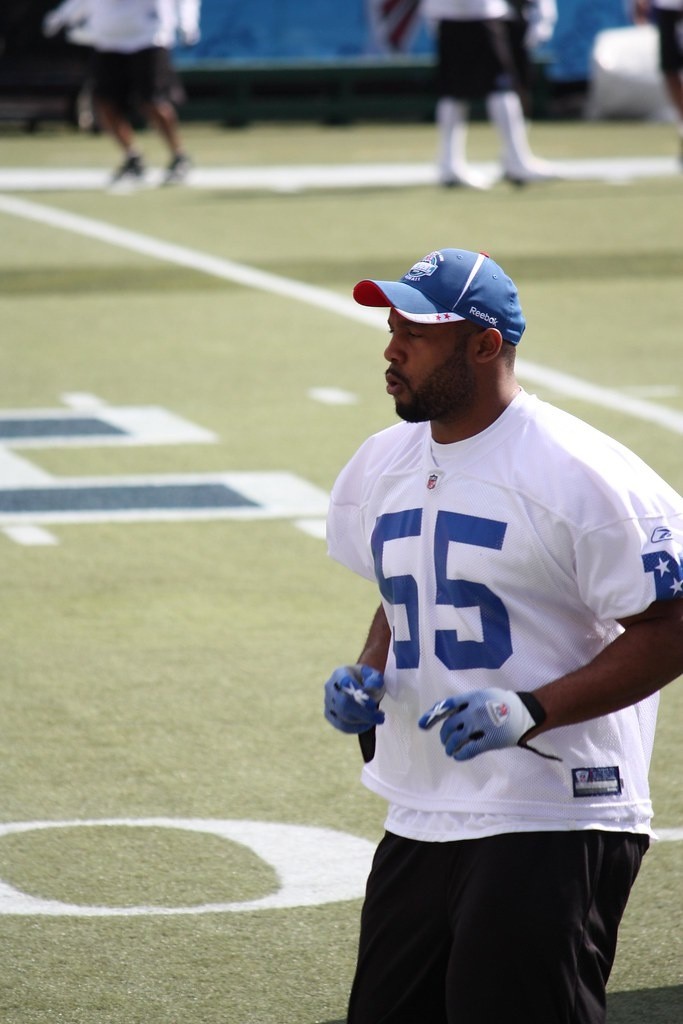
[[323, 665, 386, 734], [418, 687, 546, 762]]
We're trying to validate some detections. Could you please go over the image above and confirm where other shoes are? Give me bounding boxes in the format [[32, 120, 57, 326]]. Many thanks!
[[165, 157, 188, 188], [113, 157, 143, 187], [440, 164, 490, 189], [503, 159, 567, 185]]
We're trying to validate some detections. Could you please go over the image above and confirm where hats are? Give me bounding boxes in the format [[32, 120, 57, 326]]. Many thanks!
[[354, 248, 525, 345]]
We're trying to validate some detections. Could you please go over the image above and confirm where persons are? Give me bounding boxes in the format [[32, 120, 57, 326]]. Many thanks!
[[369, 0, 683, 193], [325, 248, 683, 1024], [43, 0, 202, 189]]
